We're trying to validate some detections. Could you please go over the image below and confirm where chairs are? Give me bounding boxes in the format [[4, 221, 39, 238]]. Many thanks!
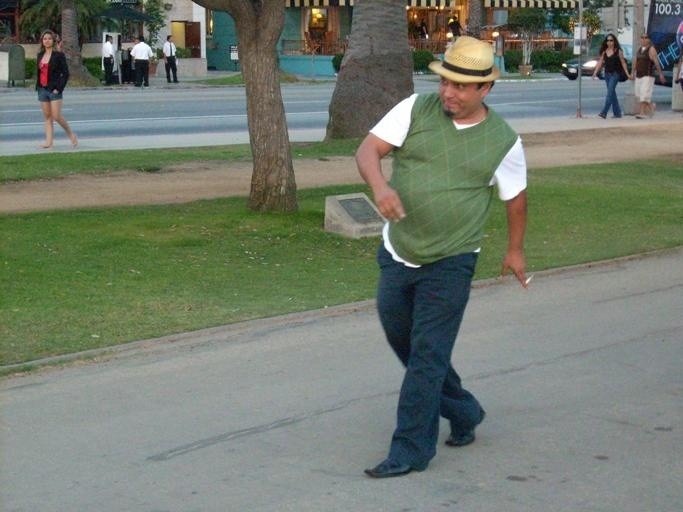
[[303, 29, 443, 55]]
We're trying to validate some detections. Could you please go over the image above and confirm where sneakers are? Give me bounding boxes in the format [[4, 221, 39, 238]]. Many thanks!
[[636, 113, 646, 119], [611, 114, 622, 118], [649, 102, 656, 118], [598, 112, 606, 119]]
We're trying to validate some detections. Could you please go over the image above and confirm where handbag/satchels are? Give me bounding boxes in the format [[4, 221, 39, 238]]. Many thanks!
[[618, 58, 628, 82]]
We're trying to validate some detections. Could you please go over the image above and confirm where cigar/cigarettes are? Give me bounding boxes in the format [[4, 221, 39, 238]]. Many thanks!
[[524, 274, 534, 285]]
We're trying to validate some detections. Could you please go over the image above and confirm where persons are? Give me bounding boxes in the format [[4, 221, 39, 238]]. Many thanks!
[[356, 36, 528, 477], [676, 54, 683, 90], [420, 17, 430, 40], [449, 16, 463, 39], [591, 34, 632, 119], [102, 36, 115, 86], [631, 33, 665, 119], [130, 36, 153, 87], [128, 39, 140, 84], [35, 29, 78, 149], [163, 35, 178, 83]]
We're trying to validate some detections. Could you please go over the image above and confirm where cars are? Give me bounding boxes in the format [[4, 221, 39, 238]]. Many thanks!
[[27, 29, 59, 43], [561, 42, 633, 82], [480, 23, 526, 40]]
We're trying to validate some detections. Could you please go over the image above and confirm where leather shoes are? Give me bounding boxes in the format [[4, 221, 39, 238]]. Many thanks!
[[445, 406, 485, 446], [364, 456, 414, 478]]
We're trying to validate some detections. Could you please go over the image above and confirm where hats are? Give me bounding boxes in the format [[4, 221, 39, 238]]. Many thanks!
[[428, 34, 499, 84]]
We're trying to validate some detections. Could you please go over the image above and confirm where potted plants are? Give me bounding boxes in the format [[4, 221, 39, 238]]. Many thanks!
[[506, 6, 551, 78]]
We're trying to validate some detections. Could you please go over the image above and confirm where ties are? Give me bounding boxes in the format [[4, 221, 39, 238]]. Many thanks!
[[170, 42, 172, 56]]
